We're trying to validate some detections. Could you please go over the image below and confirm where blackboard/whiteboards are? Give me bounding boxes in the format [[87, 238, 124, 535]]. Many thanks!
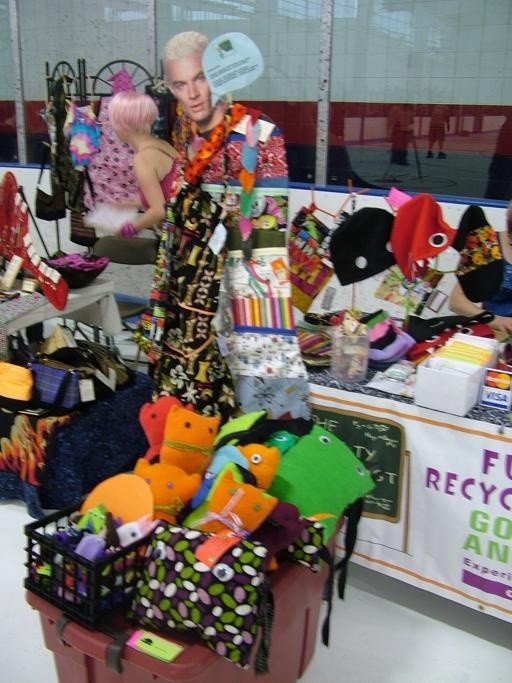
[[307, 402, 406, 523]]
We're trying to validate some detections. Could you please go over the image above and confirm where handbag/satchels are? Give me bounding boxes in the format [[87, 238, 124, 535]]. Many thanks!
[[28, 364, 81, 409], [70, 172, 97, 245], [34, 139, 66, 219], [108, 356, 154, 410]]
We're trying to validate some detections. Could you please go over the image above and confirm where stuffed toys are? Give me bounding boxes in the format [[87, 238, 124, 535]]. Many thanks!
[[78, 395, 376, 544], [34, 393, 377, 608]]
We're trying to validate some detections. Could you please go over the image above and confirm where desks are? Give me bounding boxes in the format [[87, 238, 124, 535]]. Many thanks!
[[294, 366, 512, 629]]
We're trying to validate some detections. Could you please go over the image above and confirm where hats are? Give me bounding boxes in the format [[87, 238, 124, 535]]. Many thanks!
[[451, 205, 501, 302], [405, 311, 496, 357], [391, 195, 458, 291], [329, 206, 397, 287]]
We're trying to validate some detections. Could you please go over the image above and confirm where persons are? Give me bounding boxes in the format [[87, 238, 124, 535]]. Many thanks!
[[425, 103, 449, 158], [161, 30, 291, 184], [387, 102, 416, 166], [103, 88, 188, 239], [449, 203, 511, 332]]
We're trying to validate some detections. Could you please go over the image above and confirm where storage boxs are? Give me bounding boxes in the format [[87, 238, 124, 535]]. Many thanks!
[[23, 515, 348, 683]]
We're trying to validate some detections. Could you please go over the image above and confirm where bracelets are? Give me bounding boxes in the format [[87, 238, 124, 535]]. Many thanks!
[[115, 220, 138, 239]]
